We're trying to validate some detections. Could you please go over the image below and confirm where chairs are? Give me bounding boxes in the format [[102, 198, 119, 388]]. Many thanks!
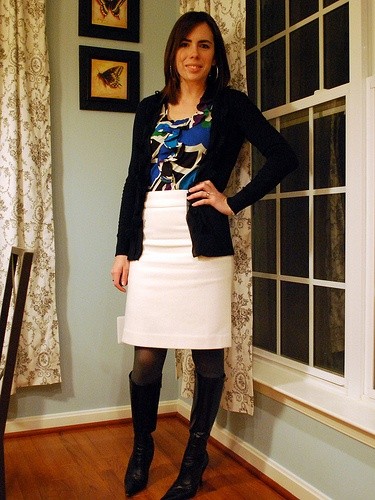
[[0, 247, 34, 500]]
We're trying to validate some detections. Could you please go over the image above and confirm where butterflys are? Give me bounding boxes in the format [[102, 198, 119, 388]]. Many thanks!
[[96, 0, 126, 20], [97, 66, 124, 88]]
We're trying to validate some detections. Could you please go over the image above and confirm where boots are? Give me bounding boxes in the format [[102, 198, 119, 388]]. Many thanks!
[[160, 370, 226, 500], [124, 370, 162, 497]]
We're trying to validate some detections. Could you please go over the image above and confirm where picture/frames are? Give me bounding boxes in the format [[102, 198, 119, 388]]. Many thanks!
[[78, 0, 141, 43], [79, 45, 141, 112]]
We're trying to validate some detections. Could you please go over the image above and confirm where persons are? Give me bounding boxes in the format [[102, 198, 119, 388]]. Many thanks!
[[112, 12, 300, 499]]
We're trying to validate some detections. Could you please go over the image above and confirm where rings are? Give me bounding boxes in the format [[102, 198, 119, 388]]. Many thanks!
[[207, 192, 210, 198], [112, 280, 114, 281]]
